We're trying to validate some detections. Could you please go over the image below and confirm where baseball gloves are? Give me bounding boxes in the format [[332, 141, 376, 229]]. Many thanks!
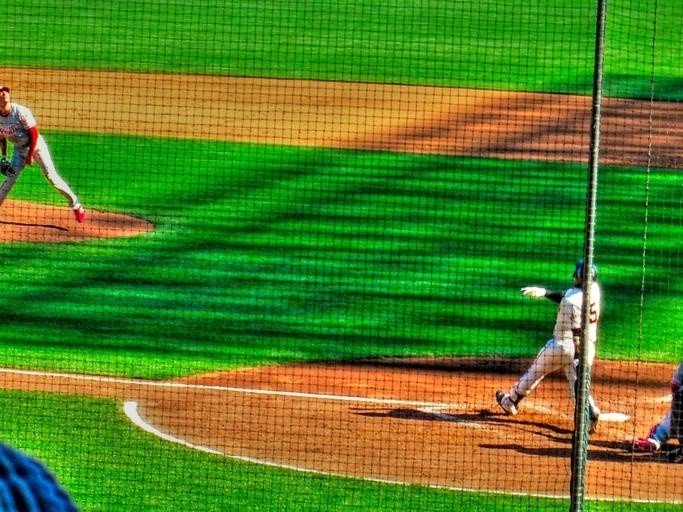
[[0, 158, 15, 176]]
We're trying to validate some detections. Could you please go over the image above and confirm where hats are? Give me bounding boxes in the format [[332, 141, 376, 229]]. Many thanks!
[[0, 86, 10, 93], [573, 260, 597, 282]]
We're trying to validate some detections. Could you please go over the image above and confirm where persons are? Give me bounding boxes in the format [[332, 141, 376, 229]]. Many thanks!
[[495, 259, 601, 434], [0, 87, 87, 222], [634, 360, 683, 463]]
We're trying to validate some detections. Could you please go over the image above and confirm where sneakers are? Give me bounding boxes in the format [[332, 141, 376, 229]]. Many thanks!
[[74, 204, 86, 223], [495, 390, 518, 416], [625, 439, 661, 452], [665, 448, 683, 463], [589, 406, 600, 435]]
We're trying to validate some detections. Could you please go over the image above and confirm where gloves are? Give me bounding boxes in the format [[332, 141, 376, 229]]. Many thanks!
[[520, 286, 546, 297]]
